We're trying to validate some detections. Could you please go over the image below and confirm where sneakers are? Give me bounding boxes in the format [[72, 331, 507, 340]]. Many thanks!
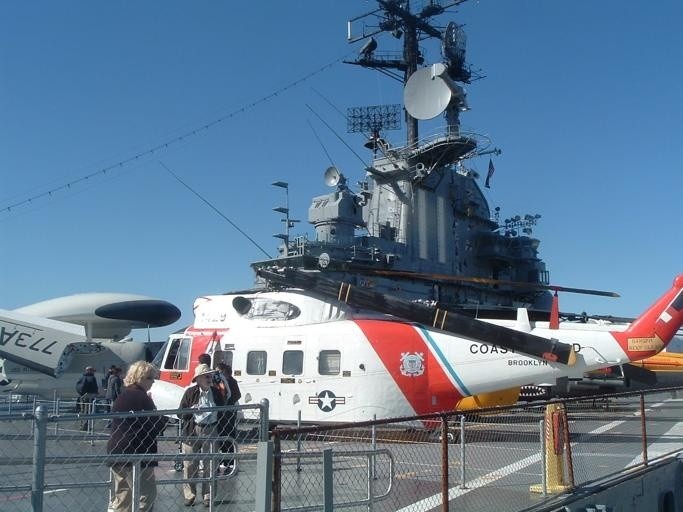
[[202, 493, 210, 507], [80, 424, 91, 431], [184, 496, 194, 506]]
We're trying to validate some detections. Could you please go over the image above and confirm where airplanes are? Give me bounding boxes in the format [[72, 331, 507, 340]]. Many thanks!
[[0, 275, 683, 445]]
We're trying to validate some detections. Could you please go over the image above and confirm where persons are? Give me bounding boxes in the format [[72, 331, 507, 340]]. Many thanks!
[[173, 363, 226, 508], [104, 358, 166, 511], [76, 352, 241, 471]]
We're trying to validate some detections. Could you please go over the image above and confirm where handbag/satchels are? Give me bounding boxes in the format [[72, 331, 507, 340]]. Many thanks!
[[75, 375, 86, 395], [211, 370, 228, 406]]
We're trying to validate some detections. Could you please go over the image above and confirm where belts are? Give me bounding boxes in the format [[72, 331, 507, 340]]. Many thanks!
[[193, 421, 218, 427]]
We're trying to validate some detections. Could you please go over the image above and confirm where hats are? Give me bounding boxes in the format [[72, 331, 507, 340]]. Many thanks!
[[191, 364, 216, 383]]
